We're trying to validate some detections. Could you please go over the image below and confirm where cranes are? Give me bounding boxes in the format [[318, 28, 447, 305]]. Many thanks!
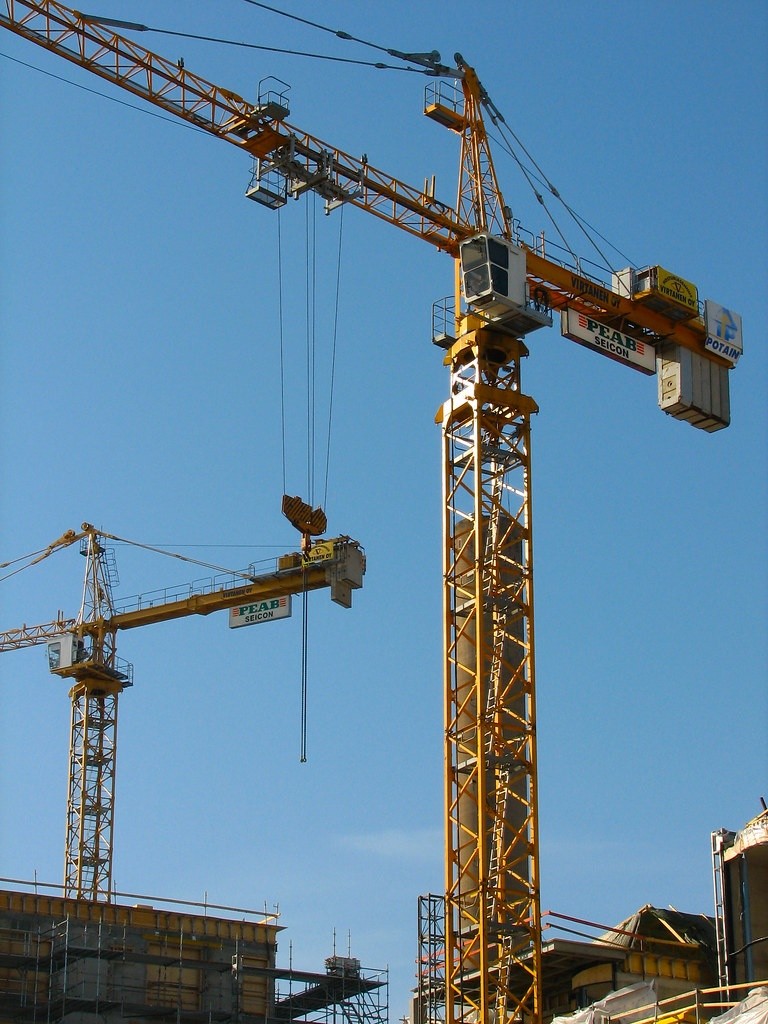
[[0, 1, 745, 1024], [3, 535, 369, 901]]
